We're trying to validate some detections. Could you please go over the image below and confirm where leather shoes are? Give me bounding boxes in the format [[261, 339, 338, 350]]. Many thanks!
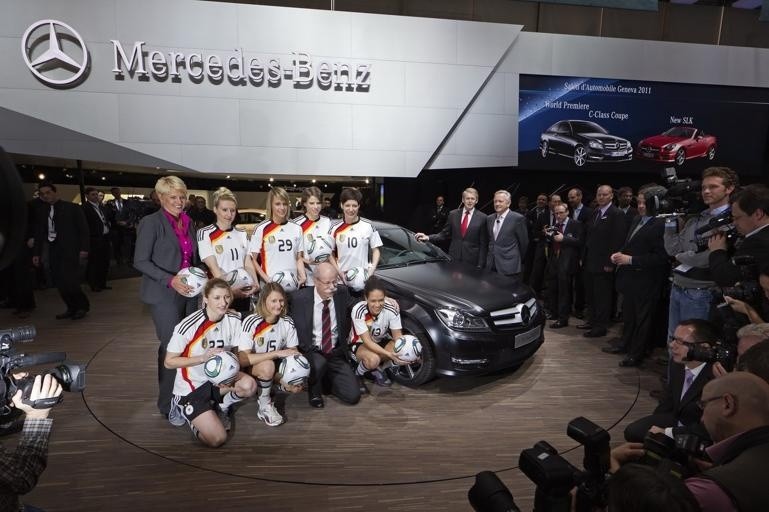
[[92, 286, 101, 293], [604, 335, 669, 400], [71, 307, 88, 320], [57, 309, 72, 319], [309, 395, 324, 409], [538, 310, 622, 337], [103, 285, 112, 289]]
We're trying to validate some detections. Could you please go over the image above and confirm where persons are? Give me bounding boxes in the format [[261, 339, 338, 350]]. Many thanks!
[[249, 186, 307, 294], [132, 171, 208, 420], [330, 188, 383, 288], [196, 186, 260, 322], [238, 282, 310, 427], [291, 186, 333, 290], [0, 179, 242, 326], [348, 275, 422, 397], [226, 261, 401, 409], [414, 166, 768, 511], [165, 277, 256, 450], [288, 196, 339, 219], [0, 370, 65, 512]]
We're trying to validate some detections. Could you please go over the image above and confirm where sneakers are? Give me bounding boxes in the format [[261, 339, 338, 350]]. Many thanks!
[[257, 401, 284, 426], [357, 374, 369, 394], [215, 403, 232, 432], [370, 366, 392, 387], [168, 395, 186, 427]]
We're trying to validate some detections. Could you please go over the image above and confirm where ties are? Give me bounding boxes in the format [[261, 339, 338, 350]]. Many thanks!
[[595, 211, 602, 225], [462, 211, 470, 236], [48, 205, 57, 242], [321, 300, 332, 355], [556, 225, 563, 250], [495, 216, 500, 239], [117, 200, 122, 212]]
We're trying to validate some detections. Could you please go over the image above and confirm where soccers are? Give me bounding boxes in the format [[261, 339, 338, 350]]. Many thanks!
[[225, 269, 254, 291], [177, 267, 208, 298], [204, 351, 240, 386], [279, 354, 311, 387], [346, 266, 368, 292], [392, 335, 423, 362], [271, 269, 298, 294], [308, 236, 332, 262]]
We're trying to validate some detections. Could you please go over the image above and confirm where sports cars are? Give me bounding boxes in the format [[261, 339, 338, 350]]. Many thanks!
[[638, 124, 721, 164]]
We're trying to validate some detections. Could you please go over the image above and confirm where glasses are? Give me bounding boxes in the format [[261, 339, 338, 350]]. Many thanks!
[[695, 394, 735, 409], [669, 335, 694, 347], [315, 277, 340, 285]]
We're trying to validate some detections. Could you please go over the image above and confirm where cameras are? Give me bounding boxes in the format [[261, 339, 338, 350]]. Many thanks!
[[468, 470, 521, 512], [710, 255, 762, 307], [636, 425, 696, 484], [545, 225, 559, 241], [566, 416, 611, 481], [682, 341, 738, 373], [519, 440, 601, 512]]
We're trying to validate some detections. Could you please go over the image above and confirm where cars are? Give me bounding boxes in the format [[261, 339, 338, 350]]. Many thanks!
[[233, 209, 273, 241], [327, 212, 551, 391], [539, 119, 634, 166]]
